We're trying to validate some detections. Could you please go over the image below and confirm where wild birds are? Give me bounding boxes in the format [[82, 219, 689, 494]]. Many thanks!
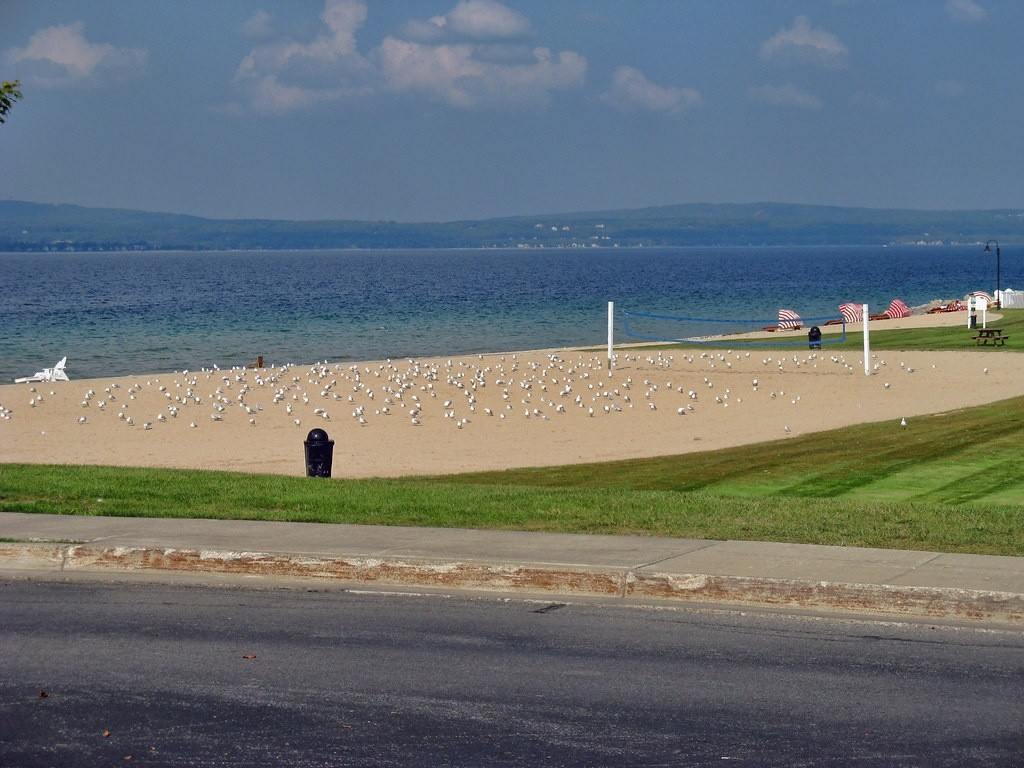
[[0, 403, 11, 422], [762, 352, 856, 373], [27, 381, 56, 408], [190, 421, 197, 429], [78, 415, 86, 424], [784, 424, 790, 432], [80, 378, 181, 430], [859, 354, 886, 370], [770, 392, 801, 405], [752, 379, 758, 391], [900, 359, 936, 373], [182, 341, 751, 431], [900, 417, 907, 430], [984, 367, 988, 373], [884, 382, 890, 389]]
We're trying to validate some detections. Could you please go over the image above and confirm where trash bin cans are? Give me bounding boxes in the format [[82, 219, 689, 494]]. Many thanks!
[[807, 326, 821, 349], [301, 429, 336, 480], [971, 315, 978, 328]]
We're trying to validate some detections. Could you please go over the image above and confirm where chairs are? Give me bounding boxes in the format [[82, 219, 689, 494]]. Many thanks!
[[823, 303, 863, 325], [926, 306, 959, 314], [869, 299, 910, 320], [762, 309, 804, 332], [960, 291, 994, 311]]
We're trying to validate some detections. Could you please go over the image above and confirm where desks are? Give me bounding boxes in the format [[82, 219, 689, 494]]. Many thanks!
[[975, 329, 1005, 347]]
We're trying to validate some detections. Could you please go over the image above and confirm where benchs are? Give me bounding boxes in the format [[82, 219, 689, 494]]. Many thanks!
[[971, 336, 987, 340], [989, 336, 1010, 340]]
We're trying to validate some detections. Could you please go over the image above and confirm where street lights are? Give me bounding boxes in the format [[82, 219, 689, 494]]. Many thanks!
[[984, 239, 1002, 312]]
